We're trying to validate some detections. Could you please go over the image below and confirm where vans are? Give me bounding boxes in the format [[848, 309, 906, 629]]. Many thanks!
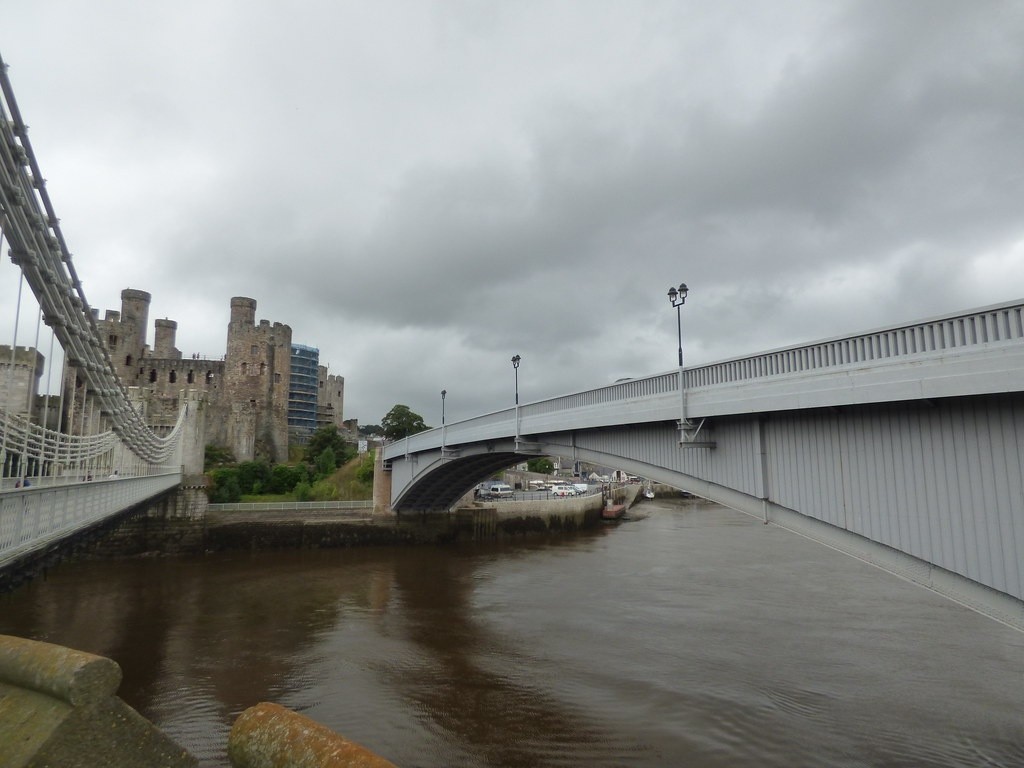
[[491, 485, 513, 497], [552, 484, 588, 495]]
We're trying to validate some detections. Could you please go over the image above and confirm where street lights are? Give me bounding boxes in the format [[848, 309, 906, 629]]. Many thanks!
[[667, 283, 690, 441], [511, 355, 523, 450], [441, 389, 446, 459]]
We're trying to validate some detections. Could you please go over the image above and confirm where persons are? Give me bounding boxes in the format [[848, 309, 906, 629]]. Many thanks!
[[15, 475, 32, 513], [192, 352, 227, 361], [109, 470, 118, 479], [83, 471, 92, 481]]
